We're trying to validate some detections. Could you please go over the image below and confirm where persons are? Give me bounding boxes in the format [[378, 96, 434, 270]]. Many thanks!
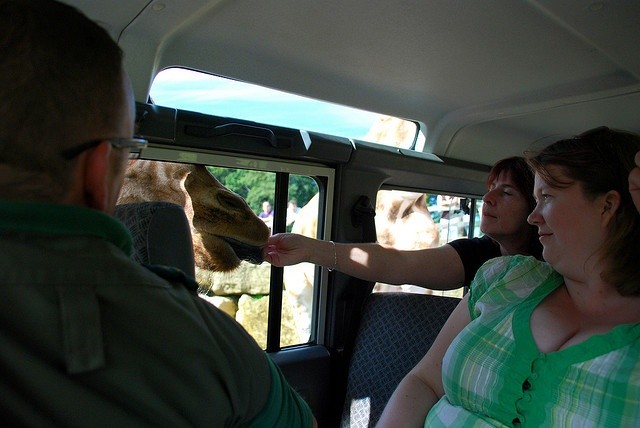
[[258, 200, 274, 219], [259, 157, 543, 299], [1, 0, 319, 428], [283, 198, 303, 223], [375, 126, 640, 428]]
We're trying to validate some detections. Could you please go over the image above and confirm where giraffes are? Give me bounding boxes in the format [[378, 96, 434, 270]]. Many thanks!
[[116, 93, 269, 272], [284, 107, 443, 348]]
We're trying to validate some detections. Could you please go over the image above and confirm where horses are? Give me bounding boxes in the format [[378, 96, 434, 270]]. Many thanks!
[[284, 190, 439, 331], [115, 156, 271, 294]]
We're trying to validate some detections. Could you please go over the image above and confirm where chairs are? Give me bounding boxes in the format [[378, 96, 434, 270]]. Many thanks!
[[341, 292, 463, 428], [118, 203, 195, 290]]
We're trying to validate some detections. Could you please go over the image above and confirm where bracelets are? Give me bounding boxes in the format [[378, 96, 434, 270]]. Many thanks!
[[327, 241, 337, 272]]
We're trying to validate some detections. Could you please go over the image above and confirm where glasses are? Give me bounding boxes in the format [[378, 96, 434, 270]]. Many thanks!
[[62, 138, 148, 160]]
